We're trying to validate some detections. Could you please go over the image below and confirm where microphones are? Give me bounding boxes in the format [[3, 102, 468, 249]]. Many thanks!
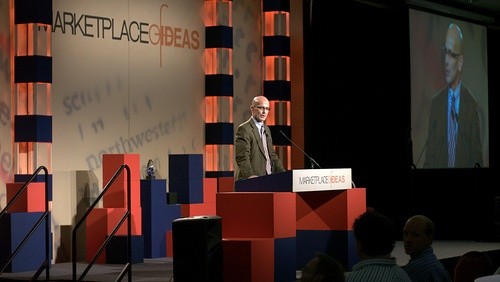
[[278, 129, 322, 168], [415, 117, 436, 167]]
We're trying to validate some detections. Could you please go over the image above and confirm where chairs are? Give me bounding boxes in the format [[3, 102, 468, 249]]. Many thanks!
[[453, 251, 494, 282]]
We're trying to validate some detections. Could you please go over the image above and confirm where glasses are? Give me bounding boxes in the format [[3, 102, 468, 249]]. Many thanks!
[[254, 105, 271, 111], [441, 47, 461, 59]]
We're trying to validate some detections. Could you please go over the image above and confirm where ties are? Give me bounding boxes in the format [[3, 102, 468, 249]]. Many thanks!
[[448, 96, 457, 168], [260, 125, 272, 175]]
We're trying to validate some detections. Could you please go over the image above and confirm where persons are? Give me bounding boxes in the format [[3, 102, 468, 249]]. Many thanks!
[[403, 214, 452, 282], [421, 22, 484, 168], [233, 95, 286, 182], [300, 251, 346, 282], [345, 211, 412, 282]]
